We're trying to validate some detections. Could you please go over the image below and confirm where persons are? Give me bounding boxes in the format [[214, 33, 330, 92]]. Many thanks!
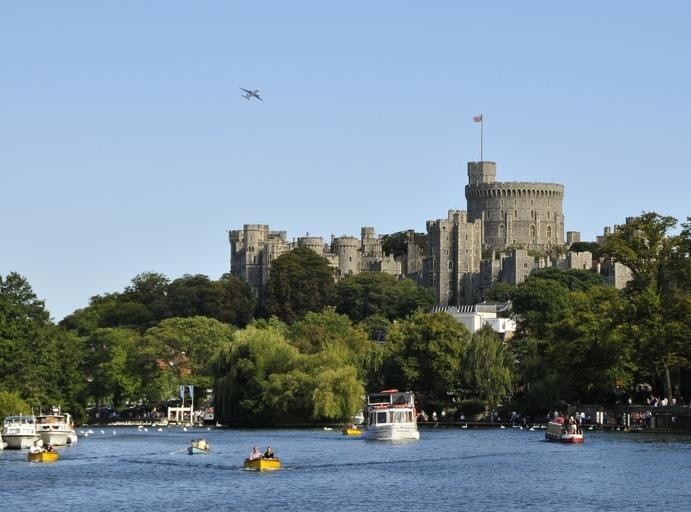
[[45, 443, 54, 452], [249, 446, 263, 459], [30, 440, 42, 454], [420, 394, 677, 434], [263, 446, 275, 459]]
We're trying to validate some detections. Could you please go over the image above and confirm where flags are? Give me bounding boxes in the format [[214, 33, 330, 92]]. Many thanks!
[[474, 116, 481, 123]]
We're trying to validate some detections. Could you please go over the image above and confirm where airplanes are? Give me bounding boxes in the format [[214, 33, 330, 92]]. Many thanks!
[[238, 87, 263, 101]]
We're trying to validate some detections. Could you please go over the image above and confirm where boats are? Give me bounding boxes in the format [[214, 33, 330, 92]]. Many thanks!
[[188, 446, 207, 454], [365, 390, 420, 442], [28, 449, 59, 463], [245, 457, 281, 471], [1, 404, 77, 450], [545, 416, 584, 444], [342, 427, 362, 435]]
